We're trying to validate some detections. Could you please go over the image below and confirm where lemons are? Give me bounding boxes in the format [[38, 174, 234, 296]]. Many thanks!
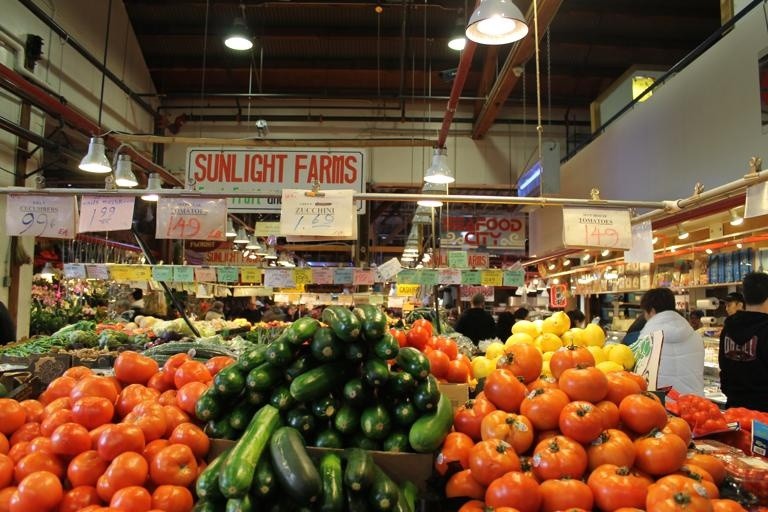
[[469, 310, 635, 390]]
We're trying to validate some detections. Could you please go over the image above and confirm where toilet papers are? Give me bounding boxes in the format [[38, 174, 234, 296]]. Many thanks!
[[700, 315, 718, 324], [696, 297, 720, 310]]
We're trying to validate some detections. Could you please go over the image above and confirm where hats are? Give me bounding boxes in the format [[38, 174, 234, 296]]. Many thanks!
[[720, 293, 743, 304]]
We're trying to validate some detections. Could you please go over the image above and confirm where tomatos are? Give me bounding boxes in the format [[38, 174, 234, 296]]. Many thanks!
[[436, 390, 747, 512], [0, 349, 237, 512], [390, 319, 469, 383]]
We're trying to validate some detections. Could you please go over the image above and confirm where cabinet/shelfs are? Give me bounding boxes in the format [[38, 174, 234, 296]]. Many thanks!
[[697, 337, 728, 411]]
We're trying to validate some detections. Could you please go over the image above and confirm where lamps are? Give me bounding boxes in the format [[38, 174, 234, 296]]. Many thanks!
[[467, 0, 528, 45], [77, 124, 277, 260], [544, 206, 744, 270], [412, 145, 453, 226], [223, 0, 256, 51], [447, 5, 467, 51]]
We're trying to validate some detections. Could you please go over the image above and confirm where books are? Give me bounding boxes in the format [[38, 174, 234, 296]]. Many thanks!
[[751, 419, 768, 458]]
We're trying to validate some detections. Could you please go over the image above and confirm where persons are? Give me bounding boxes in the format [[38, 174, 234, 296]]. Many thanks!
[[446, 292, 529, 347], [717, 272, 768, 414], [114, 300, 134, 324], [720, 291, 746, 317], [639, 287, 705, 402], [127, 289, 144, 322], [167, 297, 326, 327], [691, 310, 704, 329], [566, 308, 585, 330]]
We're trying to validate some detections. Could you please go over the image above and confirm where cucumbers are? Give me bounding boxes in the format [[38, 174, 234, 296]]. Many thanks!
[[142, 302, 455, 512]]
